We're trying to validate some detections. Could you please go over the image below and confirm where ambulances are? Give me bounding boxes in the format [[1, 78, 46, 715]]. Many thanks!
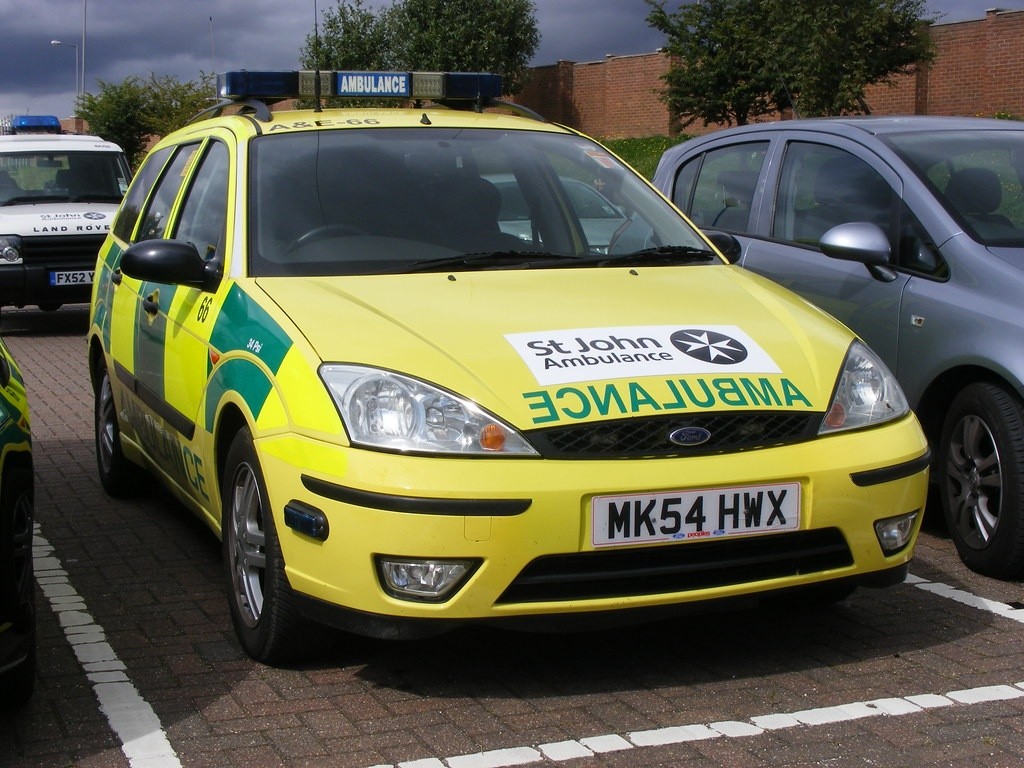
[[84, 69, 932, 663]]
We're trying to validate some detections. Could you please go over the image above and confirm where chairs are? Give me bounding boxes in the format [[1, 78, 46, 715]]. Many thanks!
[[946, 168, 1019, 241], [698, 170, 759, 232], [0, 170, 17, 187], [796, 159, 878, 241], [420, 168, 502, 248], [56, 169, 69, 191]]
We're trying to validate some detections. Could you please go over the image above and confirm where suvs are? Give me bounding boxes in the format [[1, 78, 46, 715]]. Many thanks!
[[603, 112, 1024, 583], [1, 113, 135, 314]]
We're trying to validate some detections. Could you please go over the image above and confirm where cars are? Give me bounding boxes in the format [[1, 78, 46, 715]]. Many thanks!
[[0, 341, 40, 709], [479, 169, 628, 256]]
